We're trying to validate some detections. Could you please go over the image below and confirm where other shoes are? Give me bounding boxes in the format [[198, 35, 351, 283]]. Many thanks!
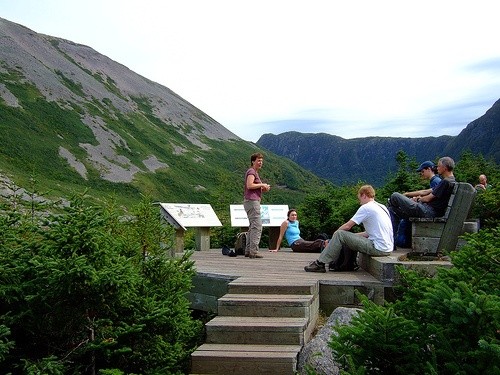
[[350, 262, 359, 271], [249, 253, 264, 258], [304, 260, 326, 272]]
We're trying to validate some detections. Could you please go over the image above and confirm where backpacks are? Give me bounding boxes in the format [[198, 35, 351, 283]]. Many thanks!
[[396, 218, 411, 248], [234, 232, 250, 255], [330, 245, 355, 270]]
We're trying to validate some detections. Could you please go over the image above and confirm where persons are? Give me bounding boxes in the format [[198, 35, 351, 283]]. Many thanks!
[[243, 152, 271, 259], [391, 160, 442, 246], [388, 157, 456, 251], [303, 185, 394, 273], [474, 174, 493, 192], [269, 209, 331, 254]]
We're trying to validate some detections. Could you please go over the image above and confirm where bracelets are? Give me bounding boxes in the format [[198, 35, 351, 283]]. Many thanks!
[[416, 198, 420, 203]]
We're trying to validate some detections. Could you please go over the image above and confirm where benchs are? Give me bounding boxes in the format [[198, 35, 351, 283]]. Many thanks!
[[408, 181, 478, 255]]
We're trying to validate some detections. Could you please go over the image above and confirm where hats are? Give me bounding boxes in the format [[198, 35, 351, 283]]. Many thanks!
[[416, 161, 434, 172]]
